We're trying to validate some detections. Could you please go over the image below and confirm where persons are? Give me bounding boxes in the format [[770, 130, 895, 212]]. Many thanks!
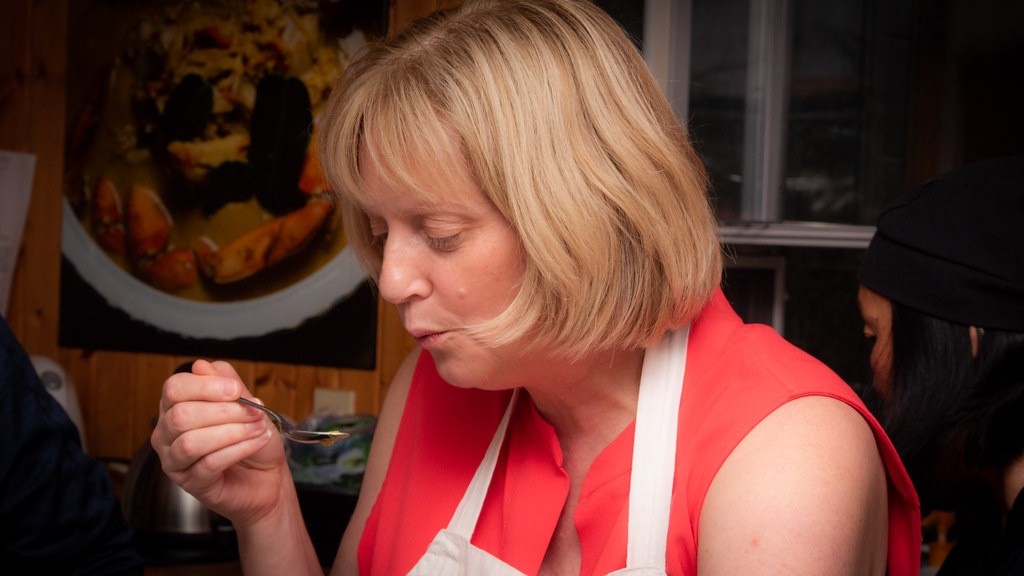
[[1, 315, 145, 575], [856, 157, 1024, 576], [151, 0, 922, 576]]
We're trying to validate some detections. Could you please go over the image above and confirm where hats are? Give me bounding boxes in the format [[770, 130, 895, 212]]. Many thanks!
[[856, 166, 1024, 332]]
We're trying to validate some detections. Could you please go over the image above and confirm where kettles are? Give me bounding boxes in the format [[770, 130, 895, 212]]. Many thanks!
[[119, 365, 232, 532]]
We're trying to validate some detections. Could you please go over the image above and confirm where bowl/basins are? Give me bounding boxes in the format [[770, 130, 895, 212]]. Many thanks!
[[56, 31, 375, 337]]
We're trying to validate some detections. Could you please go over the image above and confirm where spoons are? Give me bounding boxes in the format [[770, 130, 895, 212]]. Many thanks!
[[234, 396, 349, 443]]
